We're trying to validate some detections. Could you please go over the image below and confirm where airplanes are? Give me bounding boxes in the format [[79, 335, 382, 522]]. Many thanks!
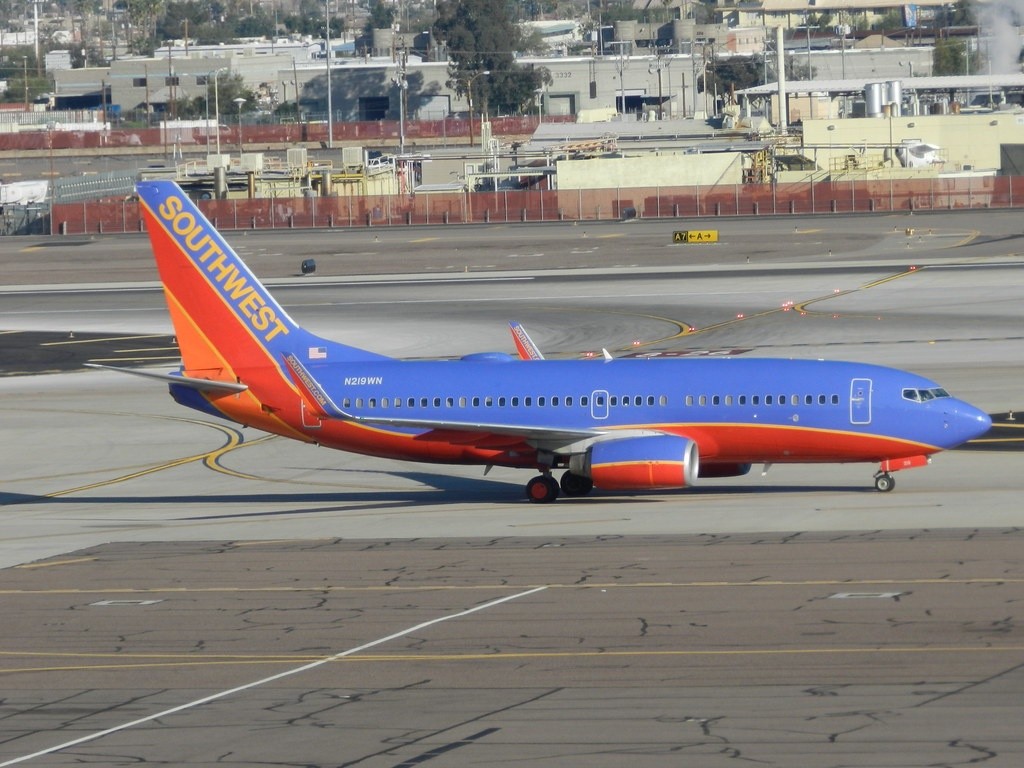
[[133, 180, 993, 504]]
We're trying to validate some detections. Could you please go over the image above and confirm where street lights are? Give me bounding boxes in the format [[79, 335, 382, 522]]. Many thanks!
[[204, 69, 217, 155], [934, 8, 958, 43], [406, 31, 431, 64], [214, 65, 228, 155], [468, 69, 491, 146], [233, 97, 246, 157]]
